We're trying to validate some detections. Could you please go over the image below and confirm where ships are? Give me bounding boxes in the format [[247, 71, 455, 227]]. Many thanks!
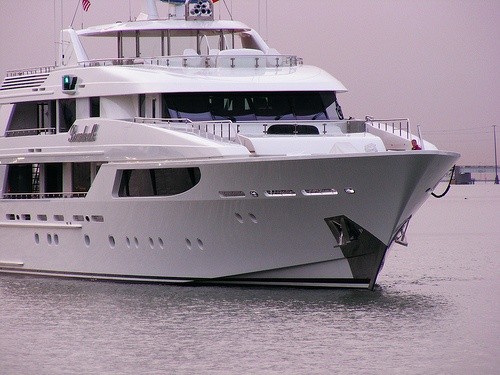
[[0, 0, 463, 292]]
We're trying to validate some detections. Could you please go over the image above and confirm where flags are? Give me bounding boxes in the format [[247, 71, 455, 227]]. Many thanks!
[[80, 0, 92, 12]]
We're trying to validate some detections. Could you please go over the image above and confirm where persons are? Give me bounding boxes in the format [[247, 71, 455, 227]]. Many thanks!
[[409, 139, 421, 151]]
